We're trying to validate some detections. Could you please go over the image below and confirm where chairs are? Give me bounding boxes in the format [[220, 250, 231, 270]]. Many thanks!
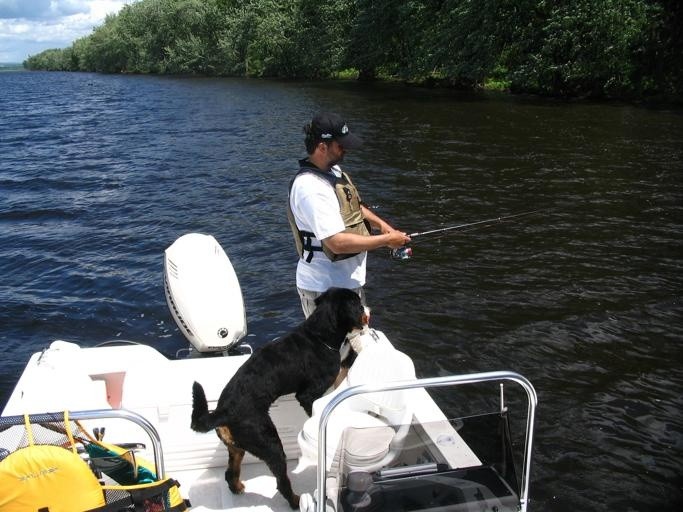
[[294, 344, 416, 475]]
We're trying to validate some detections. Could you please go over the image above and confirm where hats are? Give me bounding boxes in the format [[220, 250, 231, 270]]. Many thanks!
[[310, 111, 364, 149]]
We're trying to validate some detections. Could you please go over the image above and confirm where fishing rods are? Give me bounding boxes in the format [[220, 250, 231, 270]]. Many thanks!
[[392, 210, 546, 259]]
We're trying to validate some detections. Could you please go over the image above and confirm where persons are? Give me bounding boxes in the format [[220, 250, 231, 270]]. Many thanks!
[[286, 111, 412, 388]]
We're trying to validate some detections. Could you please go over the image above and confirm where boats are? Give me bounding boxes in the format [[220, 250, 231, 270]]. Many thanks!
[[0, 234, 539, 511]]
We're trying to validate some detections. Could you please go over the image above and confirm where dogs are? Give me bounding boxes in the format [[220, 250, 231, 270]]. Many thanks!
[[191, 287, 369, 511]]
